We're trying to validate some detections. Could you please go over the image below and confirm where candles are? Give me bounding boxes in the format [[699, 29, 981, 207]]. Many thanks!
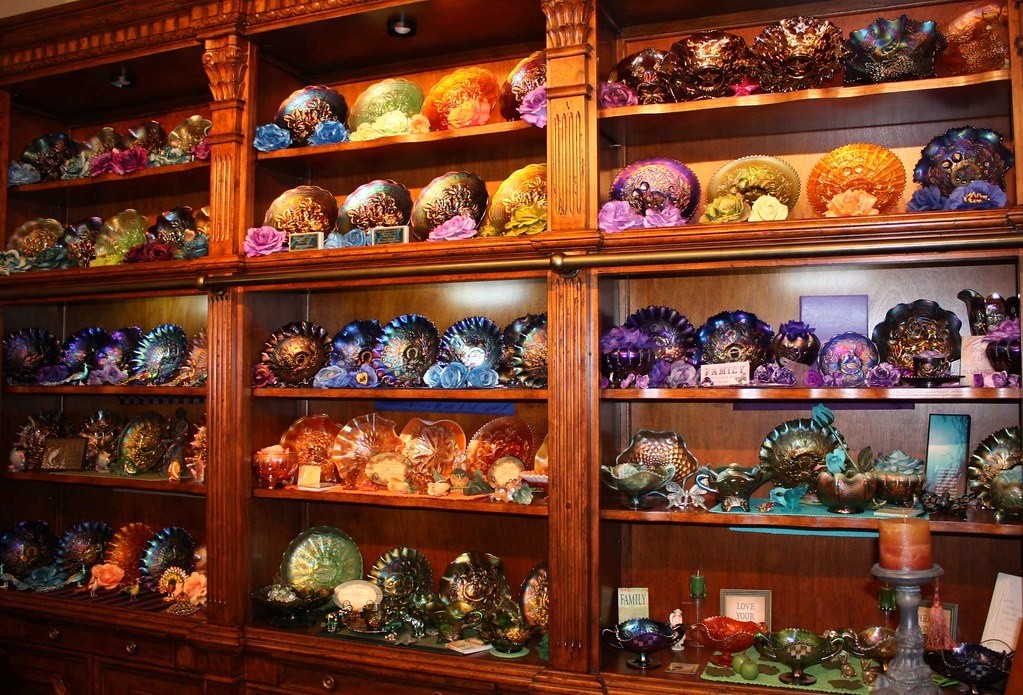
[[690, 569, 706, 599], [878, 511, 933, 571], [878, 581, 896, 610]]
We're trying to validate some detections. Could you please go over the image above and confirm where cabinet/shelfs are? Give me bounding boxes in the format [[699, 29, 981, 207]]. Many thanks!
[[0, 0, 1023, 694]]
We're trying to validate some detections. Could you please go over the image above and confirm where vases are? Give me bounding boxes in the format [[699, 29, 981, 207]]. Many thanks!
[[601, 347, 655, 379], [873, 471, 925, 506], [774, 331, 819, 370], [818, 472, 876, 515], [986, 339, 1022, 383]]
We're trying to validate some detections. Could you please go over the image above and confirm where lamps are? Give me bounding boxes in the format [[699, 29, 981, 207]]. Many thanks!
[[386, 20, 417, 38]]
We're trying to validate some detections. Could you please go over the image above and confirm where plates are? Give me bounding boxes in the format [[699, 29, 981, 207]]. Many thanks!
[[250, 524, 549, 662], [607, 285, 1022, 384], [0, 323, 210, 387], [603, 2, 1011, 107], [603, 416, 1023, 521], [264, 160, 548, 243], [280, 410, 552, 496], [273, 47, 547, 149], [609, 123, 1017, 221], [262, 309, 548, 388], [0, 515, 210, 613], [7, 204, 209, 256], [20, 114, 211, 168], [7, 408, 206, 483]]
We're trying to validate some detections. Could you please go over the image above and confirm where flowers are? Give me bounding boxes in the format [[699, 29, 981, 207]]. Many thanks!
[[324, 230, 374, 247], [31, 566, 59, 587], [503, 201, 549, 238], [89, 235, 209, 268], [903, 181, 1006, 213], [312, 364, 379, 389], [32, 245, 68, 270], [822, 190, 881, 219], [181, 571, 208, 608], [251, 361, 278, 390], [597, 317, 1022, 389], [597, 195, 790, 232], [811, 404, 875, 476], [871, 447, 926, 477], [249, 87, 548, 153], [427, 215, 479, 241], [90, 564, 124, 590], [0, 247, 31, 276], [0, 136, 210, 187], [760, 480, 822, 511], [600, 82, 640, 110], [386, 469, 536, 505], [242, 227, 289, 258], [423, 364, 500, 390]]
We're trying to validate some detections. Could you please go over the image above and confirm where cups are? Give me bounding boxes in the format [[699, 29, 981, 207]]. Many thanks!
[[603, 618, 1014, 693], [912, 354, 951, 376], [694, 463, 773, 511]]
